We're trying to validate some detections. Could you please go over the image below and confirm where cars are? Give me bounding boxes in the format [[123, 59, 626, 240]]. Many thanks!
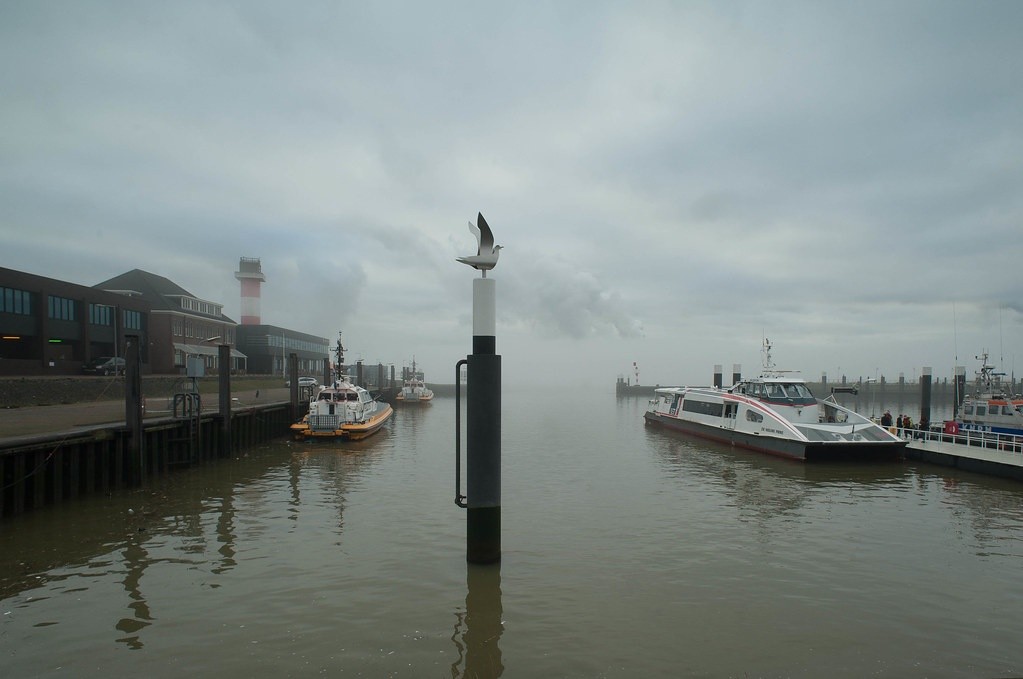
[[83, 357, 126, 376], [283, 377, 317, 388]]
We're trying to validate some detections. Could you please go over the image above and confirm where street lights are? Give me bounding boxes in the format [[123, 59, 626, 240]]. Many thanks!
[[96, 304, 118, 377]]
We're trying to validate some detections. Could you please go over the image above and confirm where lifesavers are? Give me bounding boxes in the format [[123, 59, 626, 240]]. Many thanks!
[[946, 422, 959, 434]]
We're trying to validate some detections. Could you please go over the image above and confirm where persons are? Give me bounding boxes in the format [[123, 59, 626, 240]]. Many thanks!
[[897, 415, 914, 438], [828, 416, 834, 423], [319, 393, 358, 400], [881, 410, 892, 430]]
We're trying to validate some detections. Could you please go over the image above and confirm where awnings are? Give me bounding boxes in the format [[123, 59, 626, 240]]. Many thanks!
[[172, 343, 248, 375]]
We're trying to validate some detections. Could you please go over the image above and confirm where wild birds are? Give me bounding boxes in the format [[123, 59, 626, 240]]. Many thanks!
[[454, 212, 505, 270]]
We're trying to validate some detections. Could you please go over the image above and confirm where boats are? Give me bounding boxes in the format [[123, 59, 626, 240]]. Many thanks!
[[644, 335, 910, 462], [395, 356, 435, 403], [290, 328, 393, 440], [916, 301, 1023, 437]]
